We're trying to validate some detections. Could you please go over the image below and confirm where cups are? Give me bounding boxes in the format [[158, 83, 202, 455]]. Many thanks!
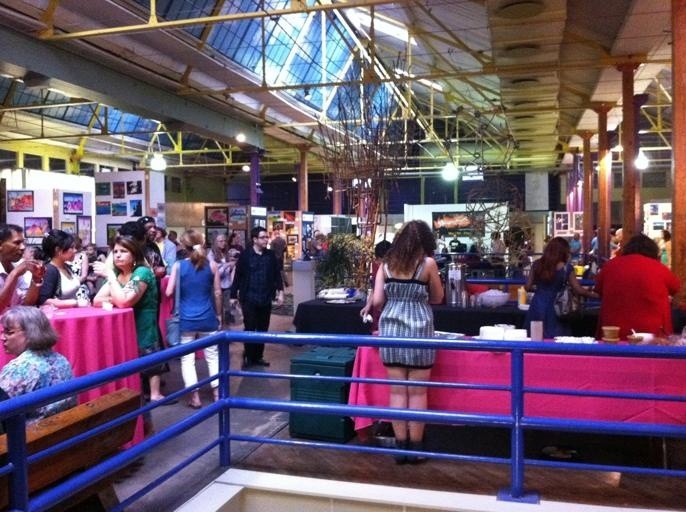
[[480, 321, 528, 341], [531, 321, 544, 342], [603, 326, 654, 345], [576, 265, 584, 276]]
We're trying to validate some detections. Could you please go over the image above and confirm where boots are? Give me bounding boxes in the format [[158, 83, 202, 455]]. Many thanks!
[[391, 439, 430, 466]]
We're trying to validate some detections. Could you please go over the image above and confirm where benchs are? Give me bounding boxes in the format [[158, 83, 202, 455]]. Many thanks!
[[0, 388, 142, 512]]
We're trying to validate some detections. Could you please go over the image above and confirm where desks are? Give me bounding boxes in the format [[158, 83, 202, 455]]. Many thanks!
[[0, 303, 146, 450], [157, 274, 176, 351], [348, 332, 685, 444], [293, 298, 372, 334]]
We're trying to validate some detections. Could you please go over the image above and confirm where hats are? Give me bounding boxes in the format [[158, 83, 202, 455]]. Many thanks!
[[134, 215, 157, 225]]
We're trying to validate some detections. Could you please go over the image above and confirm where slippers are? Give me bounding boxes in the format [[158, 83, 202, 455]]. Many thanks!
[[149, 394, 181, 407], [187, 398, 202, 409]]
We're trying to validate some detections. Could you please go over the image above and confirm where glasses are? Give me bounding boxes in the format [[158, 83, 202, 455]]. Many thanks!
[[259, 236, 269, 240], [1, 328, 25, 335]]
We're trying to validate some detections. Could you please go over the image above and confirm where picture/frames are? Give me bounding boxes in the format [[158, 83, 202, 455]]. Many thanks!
[[61, 222, 75, 236], [24, 217, 52, 238], [7, 191, 34, 212], [107, 224, 123, 245], [77, 216, 92, 249], [63, 192, 83, 214], [204, 206, 298, 247]]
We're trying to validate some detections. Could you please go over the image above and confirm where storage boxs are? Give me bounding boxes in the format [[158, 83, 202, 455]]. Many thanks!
[[290, 347, 358, 444]]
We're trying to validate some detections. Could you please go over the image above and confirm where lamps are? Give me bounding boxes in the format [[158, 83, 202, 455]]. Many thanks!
[[441, 136, 459, 181], [150, 133, 167, 170], [303, 87, 312, 102]]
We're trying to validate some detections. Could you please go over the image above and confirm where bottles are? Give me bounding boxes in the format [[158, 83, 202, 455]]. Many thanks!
[[367, 275, 373, 300], [76, 286, 91, 308], [517, 285, 535, 311]]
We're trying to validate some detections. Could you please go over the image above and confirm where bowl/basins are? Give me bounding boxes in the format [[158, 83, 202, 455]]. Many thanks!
[[479, 293, 511, 308]]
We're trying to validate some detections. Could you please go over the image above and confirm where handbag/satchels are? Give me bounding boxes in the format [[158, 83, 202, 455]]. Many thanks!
[[554, 281, 589, 318]]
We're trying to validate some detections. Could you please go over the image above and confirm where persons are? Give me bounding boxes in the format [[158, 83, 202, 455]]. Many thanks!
[[307, 230, 332, 255], [0, 304, 76, 434], [369, 218, 444, 462], [439, 226, 686, 347], [360, 241, 392, 330], [0, 210, 289, 411]]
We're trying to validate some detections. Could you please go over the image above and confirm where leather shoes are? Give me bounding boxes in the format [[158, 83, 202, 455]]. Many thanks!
[[253, 359, 271, 367], [241, 356, 253, 372]]
